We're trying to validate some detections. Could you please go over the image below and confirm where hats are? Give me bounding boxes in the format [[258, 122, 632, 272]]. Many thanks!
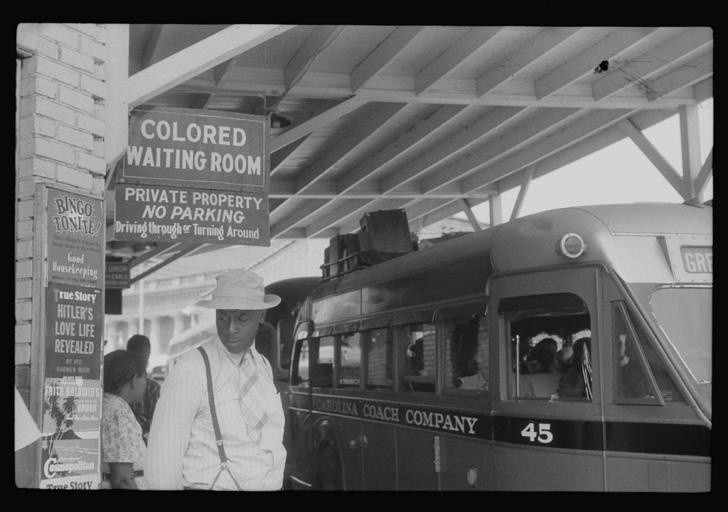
[[194, 269, 281, 310]]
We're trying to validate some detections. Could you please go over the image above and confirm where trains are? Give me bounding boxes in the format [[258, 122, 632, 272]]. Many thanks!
[[151, 202, 712, 493]]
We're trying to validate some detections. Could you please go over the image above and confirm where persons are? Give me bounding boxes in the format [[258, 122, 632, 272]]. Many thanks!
[[14, 384, 43, 488], [125, 334, 161, 447], [407, 329, 676, 405], [143, 269, 287, 491], [101, 350, 148, 490]]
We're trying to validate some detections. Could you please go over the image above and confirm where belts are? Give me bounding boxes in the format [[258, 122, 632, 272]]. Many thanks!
[[102, 470, 145, 481]]
